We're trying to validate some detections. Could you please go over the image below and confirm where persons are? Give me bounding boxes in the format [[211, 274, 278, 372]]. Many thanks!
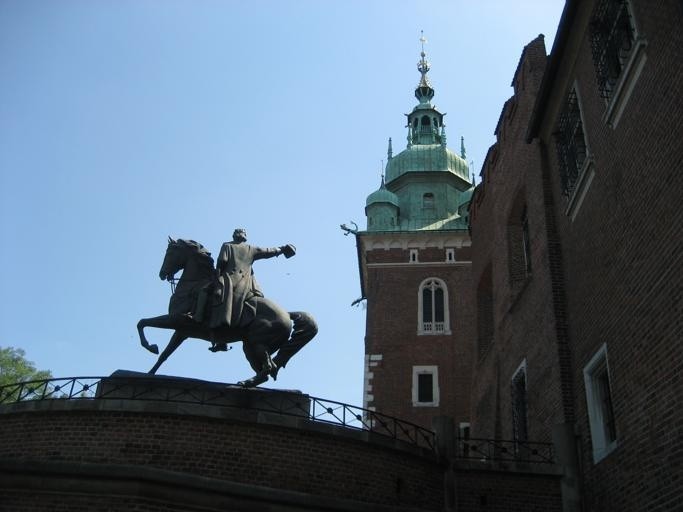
[[201, 228, 296, 352]]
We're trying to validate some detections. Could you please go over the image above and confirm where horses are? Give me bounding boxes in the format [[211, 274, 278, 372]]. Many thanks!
[[137, 236, 318, 388]]
